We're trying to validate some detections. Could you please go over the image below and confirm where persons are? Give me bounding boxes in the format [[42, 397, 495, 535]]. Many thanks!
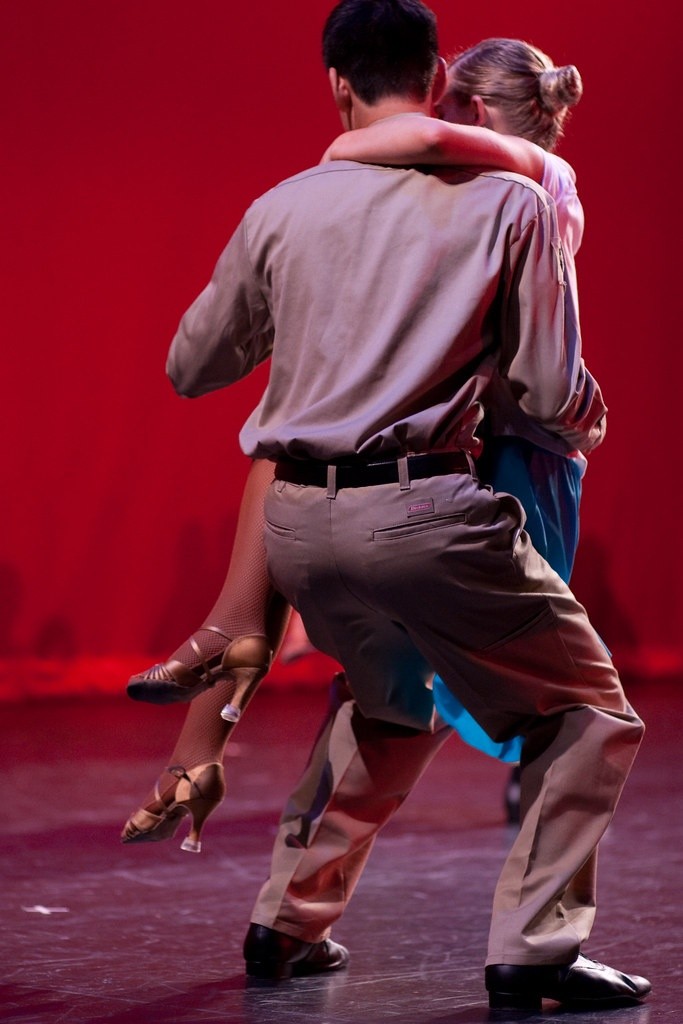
[[114, 40, 586, 852], [162, 1, 651, 1024]]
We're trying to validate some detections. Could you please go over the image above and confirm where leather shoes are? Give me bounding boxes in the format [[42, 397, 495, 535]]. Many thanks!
[[242, 922, 350, 981], [484, 951, 653, 1011]]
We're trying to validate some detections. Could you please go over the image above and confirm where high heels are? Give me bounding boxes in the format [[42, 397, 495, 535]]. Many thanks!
[[120, 762, 227, 852], [126, 624, 272, 723]]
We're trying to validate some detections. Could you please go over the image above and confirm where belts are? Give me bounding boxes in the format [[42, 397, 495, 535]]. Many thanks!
[[274, 452, 478, 489]]
